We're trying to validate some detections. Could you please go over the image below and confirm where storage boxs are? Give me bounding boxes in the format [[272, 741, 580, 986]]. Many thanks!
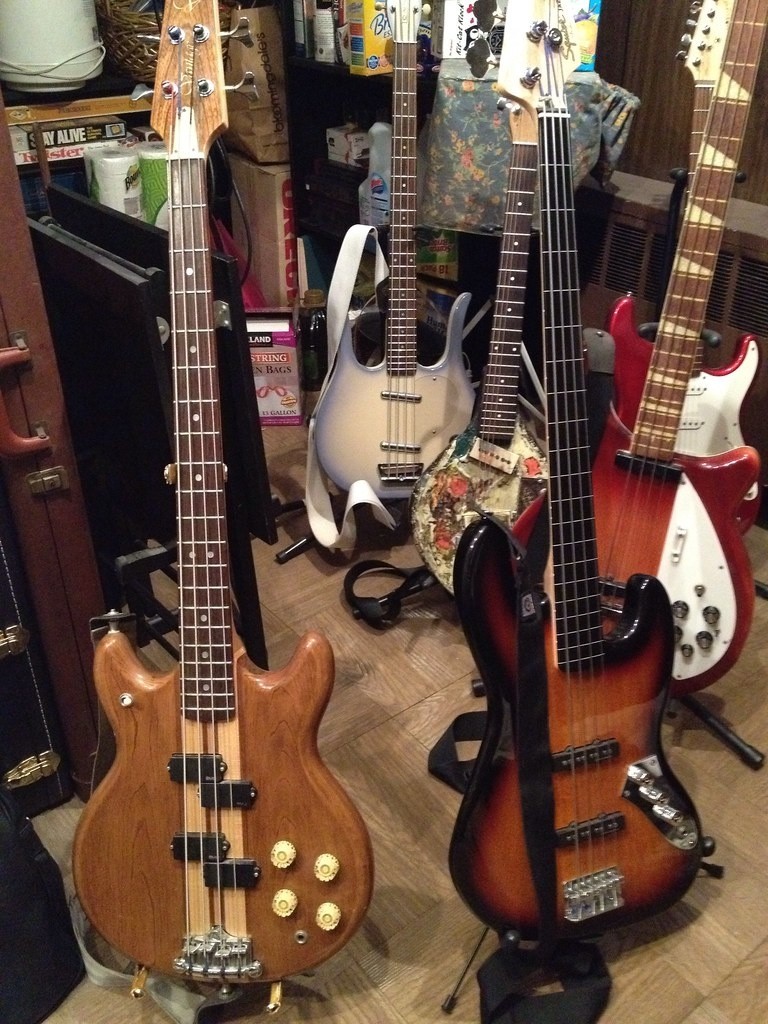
[[230, 154, 297, 307], [245, 314, 305, 425]]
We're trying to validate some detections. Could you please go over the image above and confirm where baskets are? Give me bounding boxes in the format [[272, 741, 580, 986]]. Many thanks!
[[93, 0, 232, 85]]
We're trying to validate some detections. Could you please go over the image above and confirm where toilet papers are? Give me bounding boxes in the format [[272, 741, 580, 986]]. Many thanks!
[[84, 138, 172, 236]]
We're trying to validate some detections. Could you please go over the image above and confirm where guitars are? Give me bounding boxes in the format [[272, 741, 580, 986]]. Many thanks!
[[507, 0, 764, 701], [403, 0, 556, 601], [445, 1, 718, 940], [68, 1, 378, 992], [312, 0, 480, 500]]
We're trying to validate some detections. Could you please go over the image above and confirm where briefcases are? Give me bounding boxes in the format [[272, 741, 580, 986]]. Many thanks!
[[0, 83, 117, 821]]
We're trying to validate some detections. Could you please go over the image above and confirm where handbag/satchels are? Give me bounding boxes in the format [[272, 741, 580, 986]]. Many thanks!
[[223, 1, 293, 165], [0, 789, 87, 1024]]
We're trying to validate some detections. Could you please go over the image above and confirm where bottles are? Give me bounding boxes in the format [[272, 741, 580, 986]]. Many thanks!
[[301, 290, 337, 425], [358, 121, 393, 253]]
